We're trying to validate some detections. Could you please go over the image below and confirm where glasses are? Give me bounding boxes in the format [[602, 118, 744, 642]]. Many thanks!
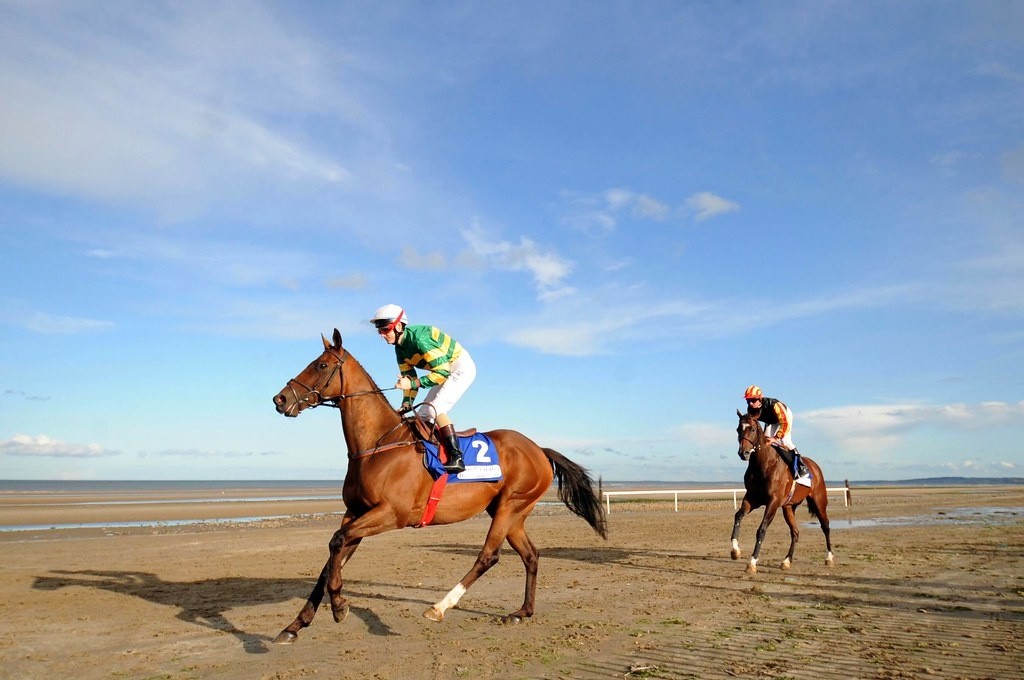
[[747, 399, 760, 403], [378, 311, 403, 334]]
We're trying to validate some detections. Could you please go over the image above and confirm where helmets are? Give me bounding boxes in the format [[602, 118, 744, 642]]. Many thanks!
[[744, 386, 762, 398], [370, 304, 407, 325]]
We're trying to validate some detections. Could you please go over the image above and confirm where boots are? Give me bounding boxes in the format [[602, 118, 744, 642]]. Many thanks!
[[789, 448, 810, 474], [439, 424, 466, 472]]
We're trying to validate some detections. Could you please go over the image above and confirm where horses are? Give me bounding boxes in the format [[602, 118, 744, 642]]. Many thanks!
[[269, 327, 608, 645], [728, 409, 834, 579]]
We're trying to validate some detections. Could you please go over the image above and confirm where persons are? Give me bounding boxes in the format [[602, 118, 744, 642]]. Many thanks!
[[369, 304, 476, 471], [744, 386, 810, 478]]
